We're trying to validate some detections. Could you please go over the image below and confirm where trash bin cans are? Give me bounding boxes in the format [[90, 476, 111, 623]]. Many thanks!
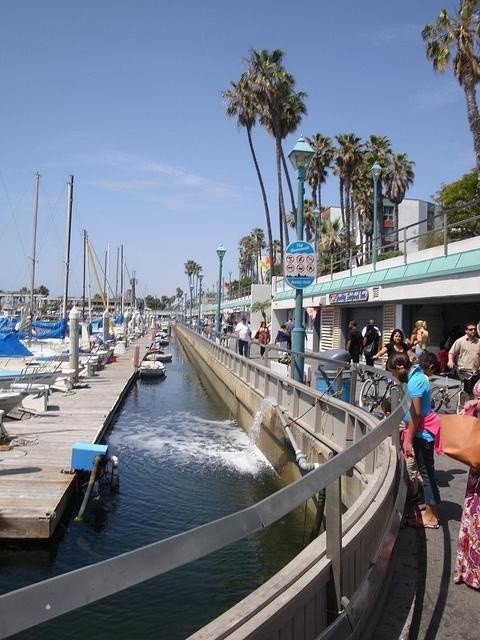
[[314, 349, 352, 402]]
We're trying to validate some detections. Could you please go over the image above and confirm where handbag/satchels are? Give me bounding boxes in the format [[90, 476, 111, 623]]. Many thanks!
[[436, 403, 480, 469]]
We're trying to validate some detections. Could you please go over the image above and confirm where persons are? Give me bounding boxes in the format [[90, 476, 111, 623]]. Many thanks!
[[446, 322, 479, 396], [223, 320, 289, 363], [385, 352, 442, 529], [455, 379, 479, 589], [203, 317, 208, 327], [347, 319, 430, 392]]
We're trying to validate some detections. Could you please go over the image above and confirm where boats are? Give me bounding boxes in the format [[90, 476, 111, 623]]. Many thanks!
[[139, 327, 172, 379]]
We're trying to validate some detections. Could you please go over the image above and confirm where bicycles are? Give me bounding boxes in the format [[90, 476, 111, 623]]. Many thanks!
[[360, 356, 405, 416], [428, 366, 479, 416], [221, 323, 232, 340]]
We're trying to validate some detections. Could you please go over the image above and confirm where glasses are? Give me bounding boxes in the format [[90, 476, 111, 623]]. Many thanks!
[[466, 327, 477, 331]]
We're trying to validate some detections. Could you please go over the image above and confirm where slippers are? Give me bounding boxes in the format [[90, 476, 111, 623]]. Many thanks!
[[403, 509, 441, 529]]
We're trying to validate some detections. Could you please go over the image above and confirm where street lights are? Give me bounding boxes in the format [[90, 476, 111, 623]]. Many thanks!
[[206, 270, 233, 303], [212, 244, 226, 339], [196, 270, 205, 333], [189, 284, 194, 326], [249, 248, 257, 284], [176, 291, 188, 323], [285, 133, 316, 384], [311, 206, 322, 258], [370, 157, 382, 270], [338, 228, 345, 271]]
